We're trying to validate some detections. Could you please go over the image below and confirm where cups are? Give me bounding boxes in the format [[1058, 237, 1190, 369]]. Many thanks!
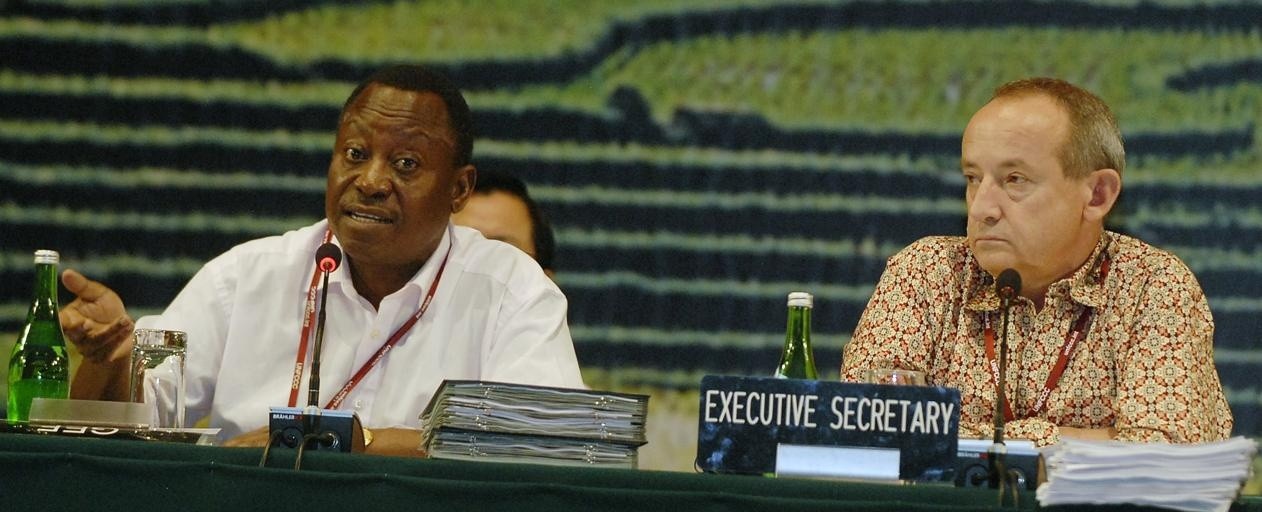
[[126, 325, 191, 441], [866, 366, 925, 387]]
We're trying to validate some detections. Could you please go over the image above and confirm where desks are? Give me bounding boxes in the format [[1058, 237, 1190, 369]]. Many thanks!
[[0, 422, 1261, 511]]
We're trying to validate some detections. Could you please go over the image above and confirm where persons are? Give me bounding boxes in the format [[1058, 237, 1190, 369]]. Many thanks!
[[56, 63, 585, 458], [833, 79, 1233, 486], [451, 171, 558, 290]]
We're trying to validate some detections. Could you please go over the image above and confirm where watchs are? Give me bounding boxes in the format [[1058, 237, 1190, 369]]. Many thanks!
[[358, 425, 372, 446]]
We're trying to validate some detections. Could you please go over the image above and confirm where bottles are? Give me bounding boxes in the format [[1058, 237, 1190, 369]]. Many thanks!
[[773, 291, 823, 379], [5, 248, 71, 425]]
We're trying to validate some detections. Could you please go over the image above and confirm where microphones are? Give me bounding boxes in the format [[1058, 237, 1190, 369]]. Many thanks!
[[268, 243, 367, 452], [957, 268, 1047, 489]]
